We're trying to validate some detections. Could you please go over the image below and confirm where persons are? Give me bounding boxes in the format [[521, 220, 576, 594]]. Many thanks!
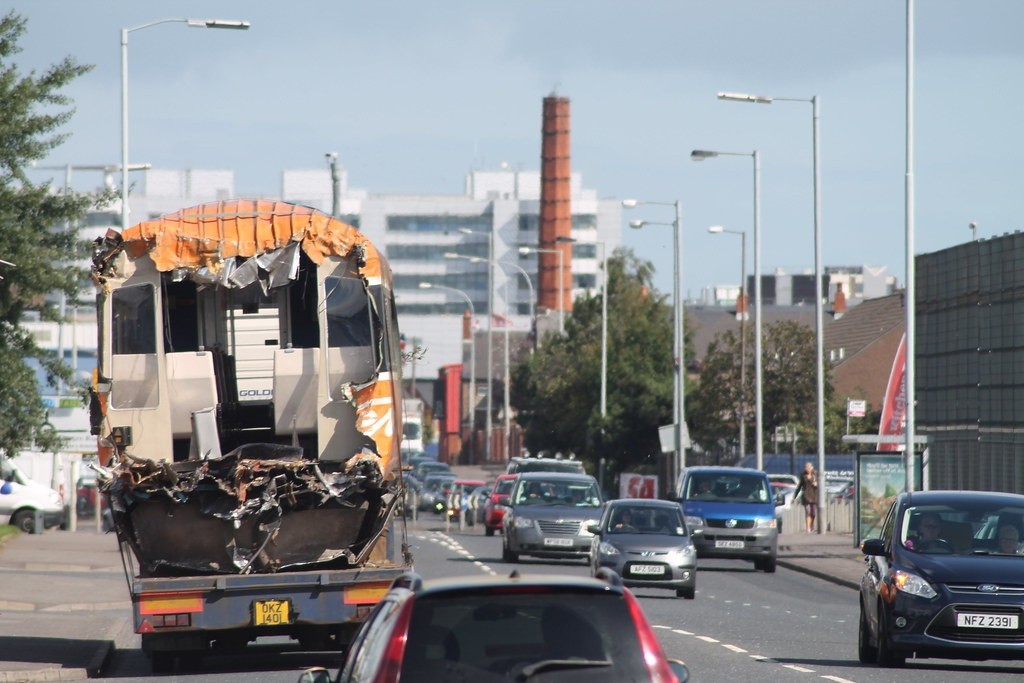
[[907, 513, 951, 550], [615, 508, 639, 533], [694, 479, 711, 497], [793, 462, 818, 533], [998, 525, 1019, 553]]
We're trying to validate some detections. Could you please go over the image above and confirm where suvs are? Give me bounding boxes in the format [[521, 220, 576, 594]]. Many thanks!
[[587, 498, 704, 599], [296, 568, 691, 683], [391, 449, 605, 562], [0, 452, 65, 534]]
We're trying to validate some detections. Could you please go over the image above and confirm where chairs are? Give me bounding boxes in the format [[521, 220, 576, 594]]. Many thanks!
[[652, 514, 671, 531]]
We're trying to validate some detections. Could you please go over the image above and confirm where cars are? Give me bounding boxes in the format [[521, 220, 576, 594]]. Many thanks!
[[859, 490, 1024, 667]]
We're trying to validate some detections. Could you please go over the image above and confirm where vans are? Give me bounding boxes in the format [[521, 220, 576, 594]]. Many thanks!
[[667, 465, 801, 573]]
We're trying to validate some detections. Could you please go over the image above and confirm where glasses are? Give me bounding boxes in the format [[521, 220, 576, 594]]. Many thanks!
[[924, 524, 937, 529], [1001, 539, 1014, 542]]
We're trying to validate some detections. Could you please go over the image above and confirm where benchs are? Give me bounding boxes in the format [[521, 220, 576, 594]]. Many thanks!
[[272, 346, 374, 436], [113, 349, 240, 439]]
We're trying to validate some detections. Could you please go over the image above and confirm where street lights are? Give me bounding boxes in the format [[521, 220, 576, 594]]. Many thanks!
[[708, 225, 746, 460], [717, 91, 827, 535], [118, 16, 251, 230], [620, 199, 685, 480], [518, 247, 564, 335], [419, 282, 475, 466], [444, 226, 535, 470], [691, 149, 764, 471], [556, 235, 609, 496]]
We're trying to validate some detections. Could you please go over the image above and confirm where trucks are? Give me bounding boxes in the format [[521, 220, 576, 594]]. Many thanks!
[[89, 196, 421, 674]]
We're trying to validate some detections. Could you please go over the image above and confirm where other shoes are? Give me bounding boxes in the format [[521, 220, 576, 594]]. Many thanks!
[[806, 528, 811, 534], [811, 526, 815, 532]]
